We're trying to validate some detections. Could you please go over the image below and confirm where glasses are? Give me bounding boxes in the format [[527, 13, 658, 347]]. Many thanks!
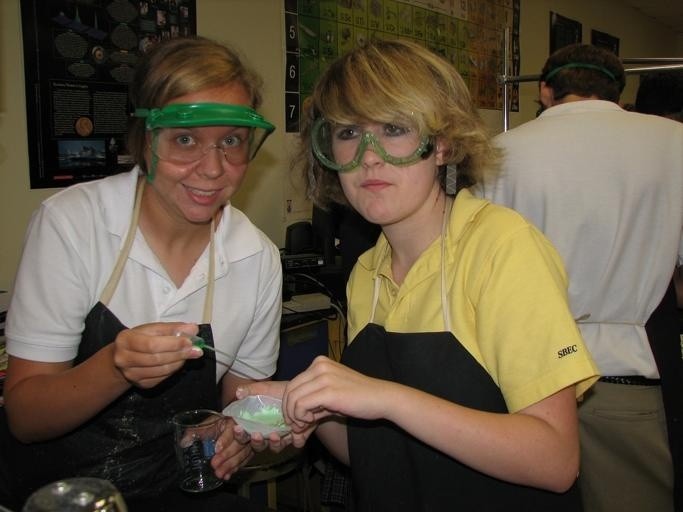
[[134, 103, 275, 165], [309, 106, 434, 173]]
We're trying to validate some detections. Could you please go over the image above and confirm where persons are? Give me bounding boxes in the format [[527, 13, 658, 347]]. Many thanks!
[[472, 40, 682, 511], [3, 34, 284, 510], [232, 34, 602, 509]]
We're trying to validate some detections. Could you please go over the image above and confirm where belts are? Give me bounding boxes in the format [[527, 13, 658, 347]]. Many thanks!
[[596, 375, 661, 385]]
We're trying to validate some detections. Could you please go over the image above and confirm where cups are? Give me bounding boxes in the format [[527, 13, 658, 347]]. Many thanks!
[[171, 410, 226, 492]]
